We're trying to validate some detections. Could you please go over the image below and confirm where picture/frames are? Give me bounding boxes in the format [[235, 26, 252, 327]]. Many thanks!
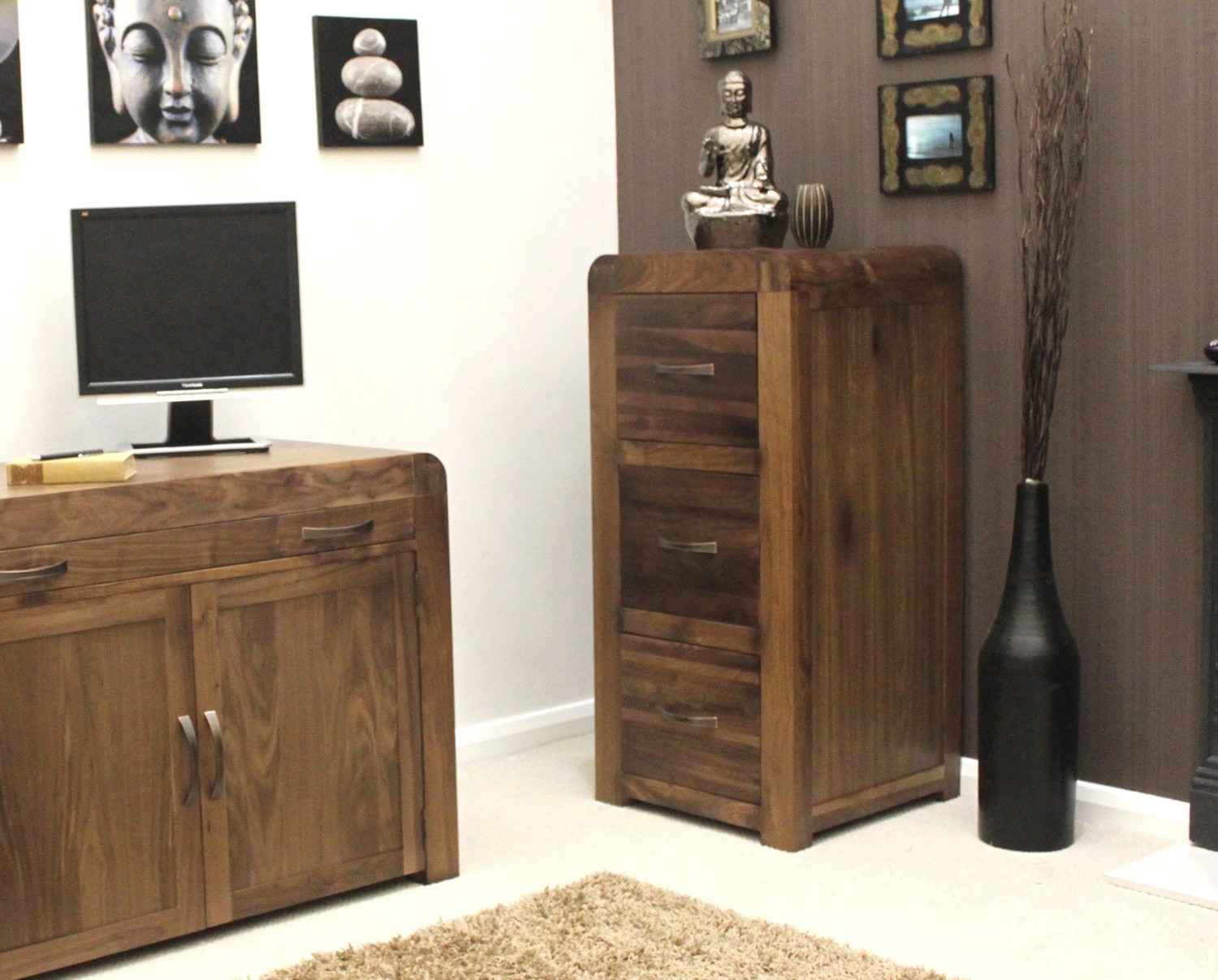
[[875, 0, 993, 58], [698, 0, 774, 60], [878, 74, 996, 195]]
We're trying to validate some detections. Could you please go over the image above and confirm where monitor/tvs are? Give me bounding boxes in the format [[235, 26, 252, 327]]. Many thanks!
[[67, 199, 306, 458]]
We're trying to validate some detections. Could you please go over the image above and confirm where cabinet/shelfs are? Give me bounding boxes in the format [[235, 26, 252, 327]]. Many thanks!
[[585, 249, 962, 850], [0, 440, 461, 979]]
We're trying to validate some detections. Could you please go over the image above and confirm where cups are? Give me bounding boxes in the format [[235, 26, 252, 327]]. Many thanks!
[[790, 183, 834, 248]]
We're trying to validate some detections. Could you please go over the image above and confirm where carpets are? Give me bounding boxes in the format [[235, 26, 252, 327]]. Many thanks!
[[265, 869, 965, 980]]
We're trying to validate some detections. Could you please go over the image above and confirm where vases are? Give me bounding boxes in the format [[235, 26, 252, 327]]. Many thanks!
[[976, 478, 1077, 848]]
